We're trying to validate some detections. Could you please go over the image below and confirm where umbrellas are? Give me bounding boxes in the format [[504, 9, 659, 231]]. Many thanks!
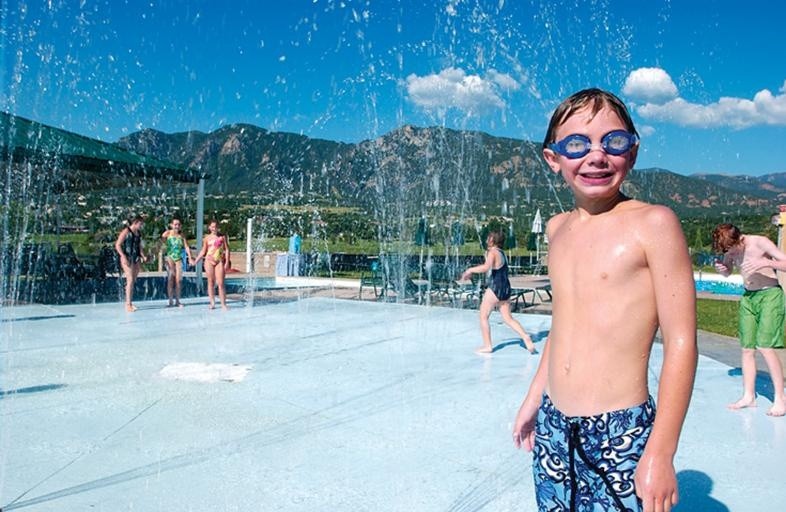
[[531, 208, 544, 262]]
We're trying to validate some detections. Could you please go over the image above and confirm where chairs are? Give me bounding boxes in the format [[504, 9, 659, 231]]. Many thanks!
[[6, 242, 117, 304], [358, 252, 551, 311]]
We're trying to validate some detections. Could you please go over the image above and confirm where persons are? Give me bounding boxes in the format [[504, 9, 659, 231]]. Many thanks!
[[370, 260, 379, 278], [459, 227, 537, 355], [711, 221, 782, 418], [503, 85, 702, 511], [151, 217, 195, 307], [194, 218, 230, 310], [113, 215, 148, 312], [288, 226, 302, 255]]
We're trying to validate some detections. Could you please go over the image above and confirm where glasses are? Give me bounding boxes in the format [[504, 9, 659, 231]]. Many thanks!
[[547, 132, 636, 159]]
